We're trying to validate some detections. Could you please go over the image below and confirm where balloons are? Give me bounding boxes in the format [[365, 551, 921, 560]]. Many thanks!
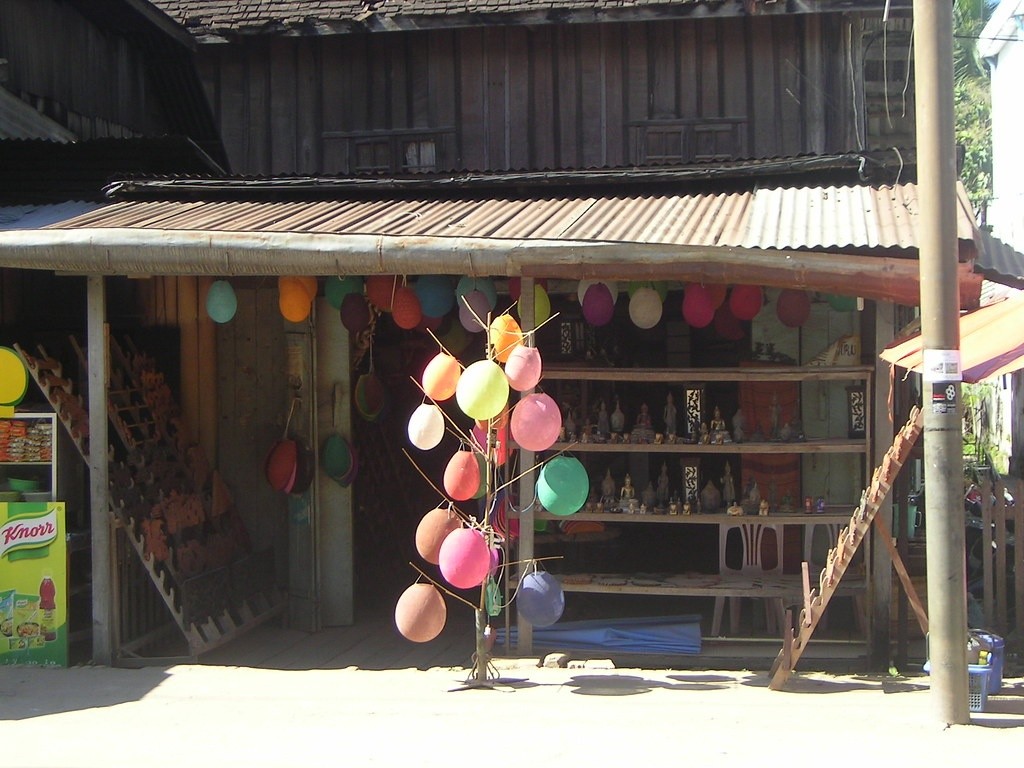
[[413, 275, 497, 334], [576, 279, 860, 329], [488, 314, 524, 363], [516, 571, 565, 627], [510, 393, 562, 452], [416, 508, 464, 565], [206, 281, 237, 323], [421, 352, 460, 401], [509, 276, 551, 327], [537, 455, 589, 515], [278, 275, 316, 322], [504, 346, 542, 391], [324, 275, 421, 332], [394, 583, 446, 643], [456, 360, 509, 420], [408, 403, 445, 450], [443, 450, 492, 501], [439, 494, 520, 589]]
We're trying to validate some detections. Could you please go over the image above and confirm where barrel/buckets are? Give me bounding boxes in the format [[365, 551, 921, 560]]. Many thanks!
[[978, 634, 1005, 695]]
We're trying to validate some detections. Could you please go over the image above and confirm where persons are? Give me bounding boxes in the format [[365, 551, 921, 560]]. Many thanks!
[[564, 391, 737, 513]]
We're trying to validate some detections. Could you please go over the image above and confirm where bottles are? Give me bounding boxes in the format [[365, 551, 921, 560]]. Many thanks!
[[39, 576, 57, 641]]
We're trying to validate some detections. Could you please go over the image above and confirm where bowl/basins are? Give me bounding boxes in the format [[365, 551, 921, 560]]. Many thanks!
[[22, 492, 51, 502], [6, 477, 38, 493], [0, 491, 21, 502]]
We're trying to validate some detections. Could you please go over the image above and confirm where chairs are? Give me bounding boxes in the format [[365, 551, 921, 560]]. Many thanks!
[[710, 522, 786, 636], [801, 522, 866, 639]]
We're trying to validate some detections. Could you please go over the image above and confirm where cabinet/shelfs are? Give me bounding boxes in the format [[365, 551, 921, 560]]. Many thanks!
[[535, 359, 874, 663]]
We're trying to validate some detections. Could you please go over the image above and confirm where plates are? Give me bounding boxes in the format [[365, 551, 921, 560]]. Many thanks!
[[355, 373, 385, 422], [266, 436, 314, 496], [323, 431, 357, 487]]
